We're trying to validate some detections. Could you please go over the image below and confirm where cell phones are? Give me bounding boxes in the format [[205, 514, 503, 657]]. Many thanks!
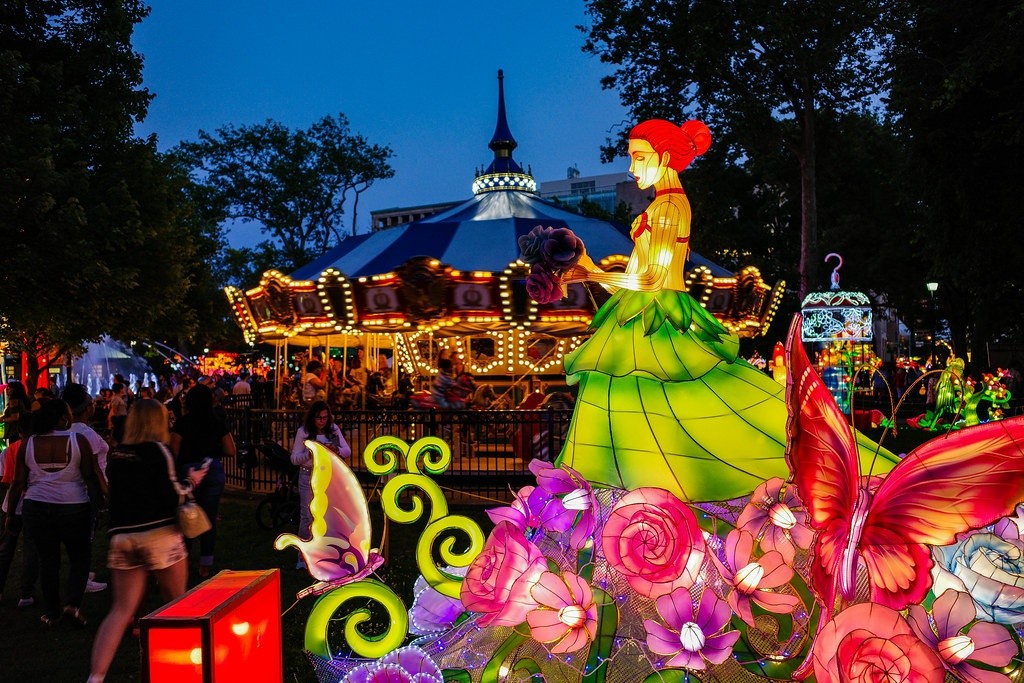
[[324, 442, 333, 446]]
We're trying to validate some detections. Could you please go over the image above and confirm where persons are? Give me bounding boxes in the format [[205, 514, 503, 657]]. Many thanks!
[[0, 378, 106, 630], [88, 399, 227, 683], [169, 386, 235, 584], [109, 362, 229, 434], [232, 352, 486, 426], [291, 403, 354, 569]]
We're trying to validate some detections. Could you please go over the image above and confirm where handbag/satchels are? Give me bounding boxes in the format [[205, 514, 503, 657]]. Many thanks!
[[176, 486, 212, 539]]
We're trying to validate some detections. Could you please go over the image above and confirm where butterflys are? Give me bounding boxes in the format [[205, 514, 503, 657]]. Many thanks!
[[273, 439, 387, 600], [784, 313, 1024, 611]]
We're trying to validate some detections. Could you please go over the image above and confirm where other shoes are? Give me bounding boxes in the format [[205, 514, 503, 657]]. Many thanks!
[[88, 571, 96, 581], [197, 571, 210, 584], [63, 604, 88, 625], [83, 578, 108, 594], [296, 558, 309, 570], [17, 597, 35, 608], [40, 614, 58, 625]]
